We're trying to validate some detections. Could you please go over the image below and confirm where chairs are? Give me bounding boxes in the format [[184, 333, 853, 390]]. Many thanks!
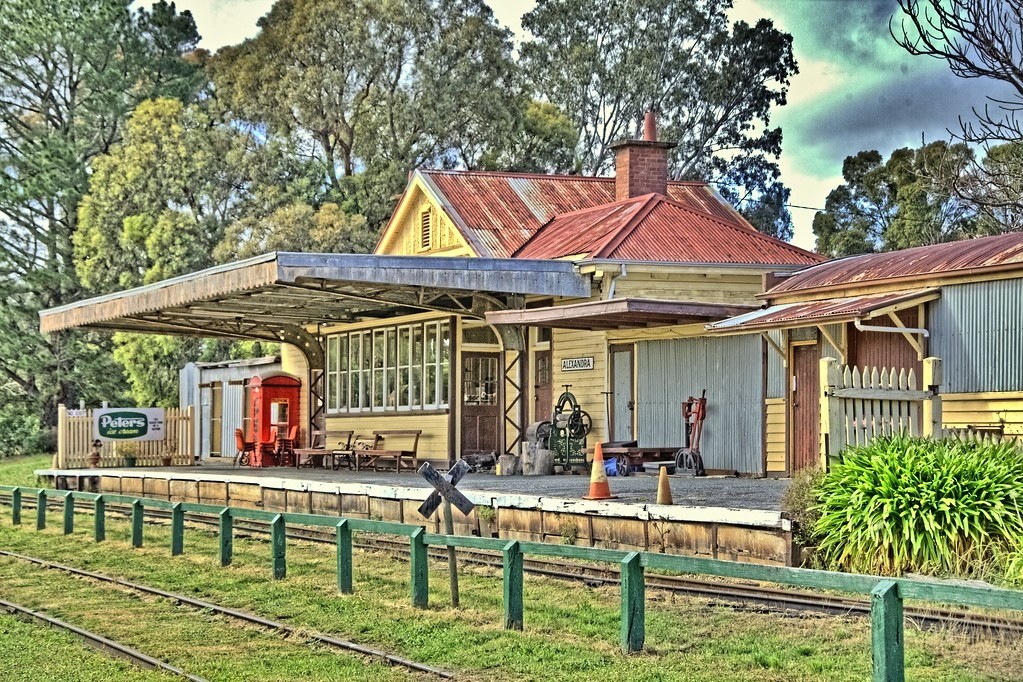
[[257, 429, 279, 468], [276, 426, 299, 468], [233, 428, 257, 468]]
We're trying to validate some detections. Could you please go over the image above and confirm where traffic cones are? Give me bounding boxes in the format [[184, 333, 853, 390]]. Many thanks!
[[582, 442, 618, 500], [656, 466, 673, 504]]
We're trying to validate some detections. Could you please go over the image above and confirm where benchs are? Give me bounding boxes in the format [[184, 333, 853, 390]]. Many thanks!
[[292, 430, 355, 470], [355, 429, 423, 475]]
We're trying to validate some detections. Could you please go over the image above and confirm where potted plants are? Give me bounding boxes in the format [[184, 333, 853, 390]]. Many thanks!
[[158, 439, 179, 466], [117, 441, 140, 467], [87, 451, 101, 468]]
